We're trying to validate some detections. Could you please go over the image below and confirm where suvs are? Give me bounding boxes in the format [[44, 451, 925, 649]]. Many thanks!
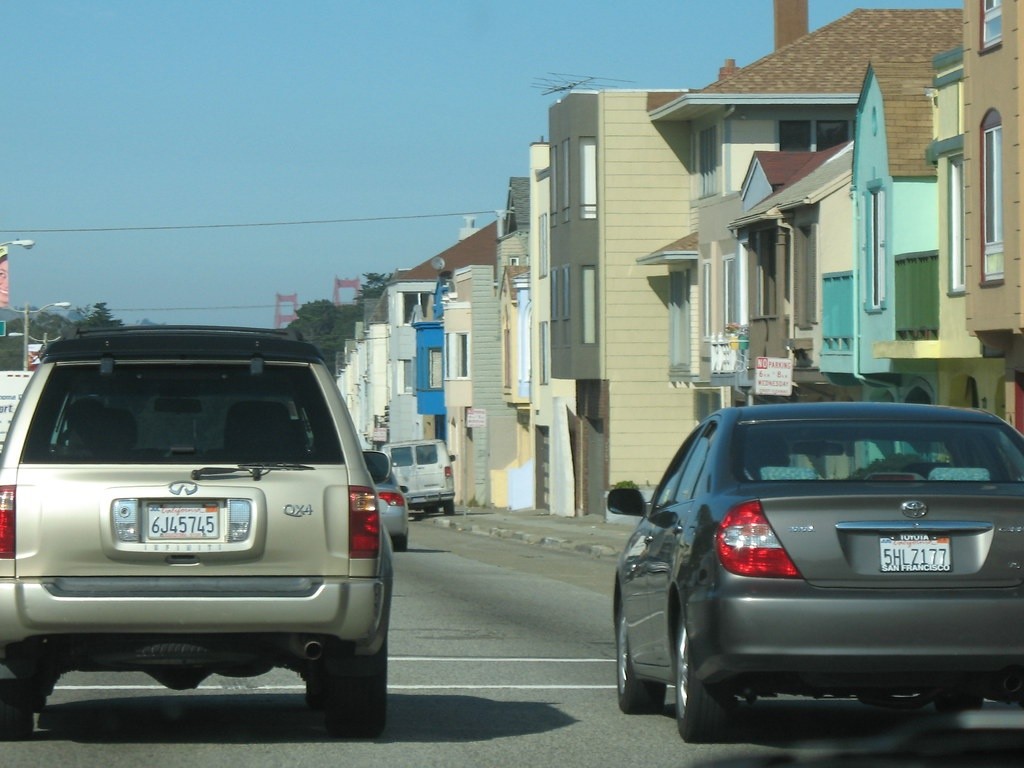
[[377, 439, 456, 519], [0, 321, 393, 737]]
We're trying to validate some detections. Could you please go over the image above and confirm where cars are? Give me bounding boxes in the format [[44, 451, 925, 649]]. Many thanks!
[[608, 402, 1024, 744], [359, 448, 410, 552]]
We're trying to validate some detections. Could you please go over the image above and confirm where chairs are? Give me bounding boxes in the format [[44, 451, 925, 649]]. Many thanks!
[[72, 409, 137, 459], [224, 399, 301, 461]]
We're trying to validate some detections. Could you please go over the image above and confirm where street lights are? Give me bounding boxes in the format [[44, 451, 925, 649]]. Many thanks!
[[8, 329, 62, 353], [1, 300, 74, 373]]
